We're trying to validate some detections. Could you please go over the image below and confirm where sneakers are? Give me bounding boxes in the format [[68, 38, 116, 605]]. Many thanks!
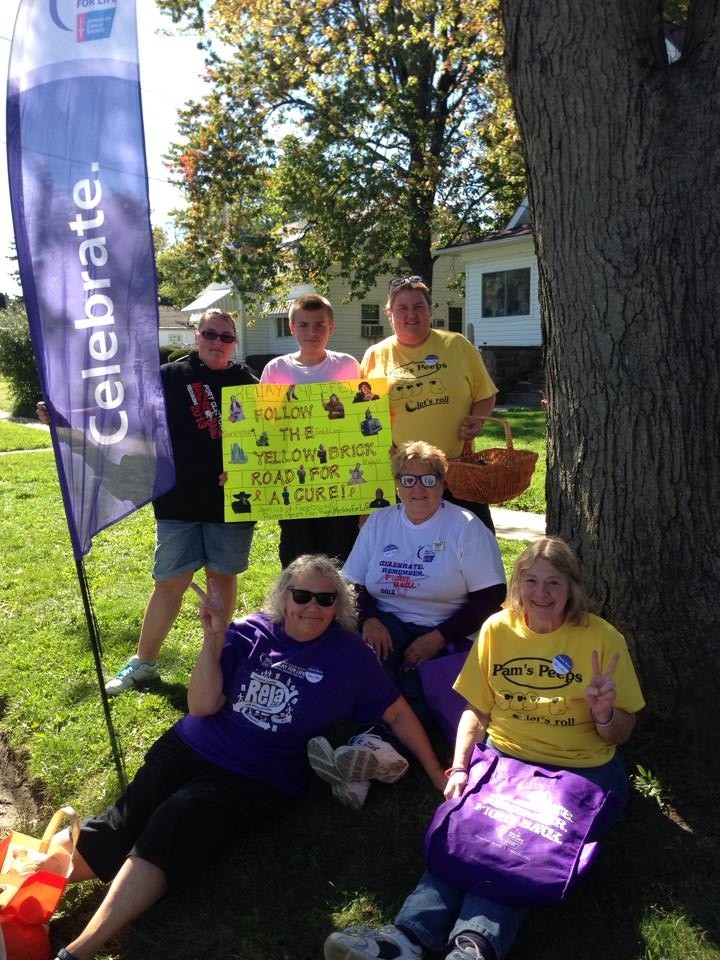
[[307, 736, 371, 811], [334, 736, 410, 785], [445, 935, 485, 960], [104, 654, 160, 696], [323, 923, 423, 960]]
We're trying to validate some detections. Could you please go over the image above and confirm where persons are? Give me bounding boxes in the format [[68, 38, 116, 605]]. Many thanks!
[[321, 393, 345, 419], [228, 395, 246, 423], [0, 550, 448, 959], [356, 273, 499, 536], [296, 465, 306, 484], [369, 488, 390, 508], [281, 487, 290, 505], [318, 561, 650, 960], [306, 437, 506, 809], [317, 443, 327, 464], [34, 310, 259, 691], [237, 294, 395, 570], [347, 463, 367, 486], [232, 492, 252, 514], [358, 382, 381, 401]]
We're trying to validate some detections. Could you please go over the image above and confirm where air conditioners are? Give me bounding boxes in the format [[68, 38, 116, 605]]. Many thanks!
[[362, 324, 385, 337], [432, 319, 445, 329]]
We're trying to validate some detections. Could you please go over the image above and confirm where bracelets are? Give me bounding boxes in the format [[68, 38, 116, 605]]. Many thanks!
[[444, 767, 468, 777], [588, 705, 616, 726]]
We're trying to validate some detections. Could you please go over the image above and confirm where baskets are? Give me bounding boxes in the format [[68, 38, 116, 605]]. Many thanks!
[[444, 416, 539, 505]]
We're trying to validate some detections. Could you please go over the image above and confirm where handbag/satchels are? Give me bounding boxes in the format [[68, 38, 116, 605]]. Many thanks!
[[0, 806, 80, 960], [424, 741, 615, 910]]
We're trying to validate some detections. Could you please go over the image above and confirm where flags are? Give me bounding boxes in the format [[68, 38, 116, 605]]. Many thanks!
[[7, 0, 177, 554]]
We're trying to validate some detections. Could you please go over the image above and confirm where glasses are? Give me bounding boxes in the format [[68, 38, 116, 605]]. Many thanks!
[[398, 473, 440, 488], [202, 330, 237, 344], [388, 275, 423, 295], [286, 587, 337, 607]]
